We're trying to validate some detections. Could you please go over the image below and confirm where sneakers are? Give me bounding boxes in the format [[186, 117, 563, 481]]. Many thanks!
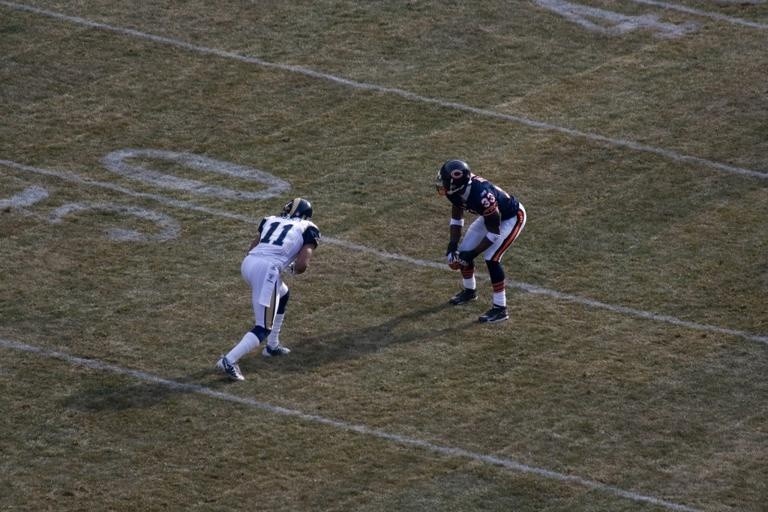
[[216, 356, 245, 381], [448, 287, 478, 305], [479, 304, 509, 324], [263, 345, 290, 357]]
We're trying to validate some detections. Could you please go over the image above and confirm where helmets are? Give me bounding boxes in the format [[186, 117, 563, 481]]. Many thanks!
[[436, 160, 471, 195], [282, 198, 312, 220]]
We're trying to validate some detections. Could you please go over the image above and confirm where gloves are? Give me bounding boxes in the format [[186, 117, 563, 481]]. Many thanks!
[[454, 250, 476, 266], [446, 242, 457, 256], [286, 261, 298, 276]]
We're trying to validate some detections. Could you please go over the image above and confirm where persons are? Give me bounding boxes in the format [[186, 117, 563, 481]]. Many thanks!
[[436, 158, 528, 323], [213, 197, 322, 382]]
